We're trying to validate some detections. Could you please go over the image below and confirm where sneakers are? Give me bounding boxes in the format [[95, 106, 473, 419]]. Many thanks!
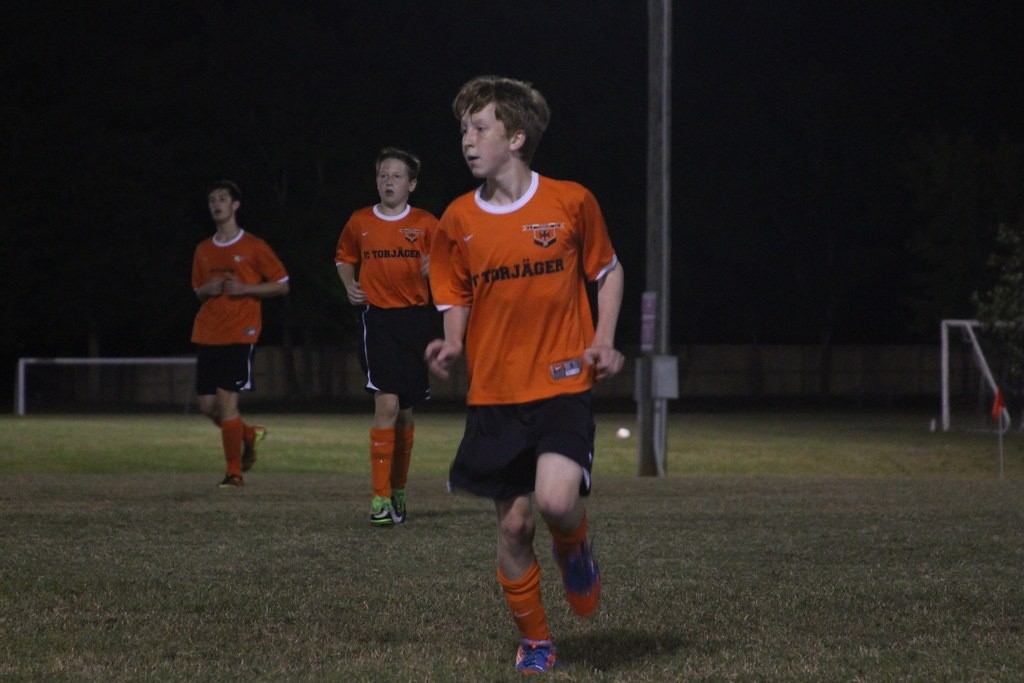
[[218, 475, 246, 489], [242, 450, 256, 473], [552, 544, 602, 619], [392, 489, 407, 524], [368, 494, 392, 527], [515, 637, 558, 676]]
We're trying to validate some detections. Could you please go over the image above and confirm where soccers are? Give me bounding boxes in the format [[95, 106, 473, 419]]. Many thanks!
[[617, 428, 630, 439]]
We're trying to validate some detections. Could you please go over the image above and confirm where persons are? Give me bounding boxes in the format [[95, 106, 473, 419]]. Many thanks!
[[335, 149, 439, 526], [423, 73, 626, 673], [191, 180, 287, 488]]
